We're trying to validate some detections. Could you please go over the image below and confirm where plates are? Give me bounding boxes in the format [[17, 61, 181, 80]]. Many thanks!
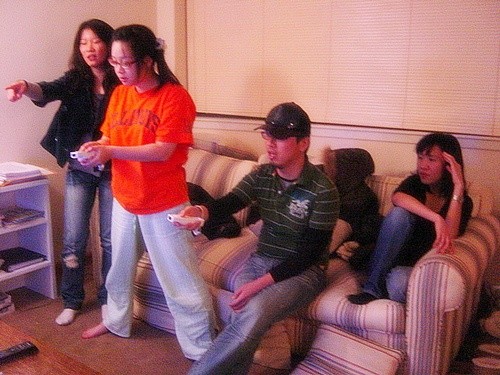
[[477, 344, 500, 355]]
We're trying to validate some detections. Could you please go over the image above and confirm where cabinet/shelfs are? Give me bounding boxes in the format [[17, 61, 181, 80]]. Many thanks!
[[0, 163, 58, 299]]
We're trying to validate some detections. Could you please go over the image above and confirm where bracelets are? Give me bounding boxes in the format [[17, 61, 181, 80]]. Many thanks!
[[22, 80, 29, 95]]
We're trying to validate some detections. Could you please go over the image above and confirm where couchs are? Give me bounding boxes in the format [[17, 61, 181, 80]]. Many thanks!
[[90, 138, 499, 374]]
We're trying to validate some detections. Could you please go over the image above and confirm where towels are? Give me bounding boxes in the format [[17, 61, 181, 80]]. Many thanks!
[[0, 162, 40, 181]]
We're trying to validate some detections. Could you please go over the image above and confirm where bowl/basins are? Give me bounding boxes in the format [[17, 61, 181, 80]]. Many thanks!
[[470, 357, 500, 375]]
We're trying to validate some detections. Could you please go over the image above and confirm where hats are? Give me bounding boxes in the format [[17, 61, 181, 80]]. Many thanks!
[[253, 102, 312, 139]]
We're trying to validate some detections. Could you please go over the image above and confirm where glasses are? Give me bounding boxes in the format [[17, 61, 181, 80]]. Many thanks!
[[260, 129, 279, 141], [109, 57, 137, 68]]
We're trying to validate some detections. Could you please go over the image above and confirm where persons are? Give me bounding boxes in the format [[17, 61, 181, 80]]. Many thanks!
[[4, 19, 117, 327], [78, 24, 216, 364], [345, 133, 474, 304], [174, 102, 340, 375]]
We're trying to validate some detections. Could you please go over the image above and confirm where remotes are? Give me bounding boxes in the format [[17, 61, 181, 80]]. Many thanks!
[[1, 341, 39, 363]]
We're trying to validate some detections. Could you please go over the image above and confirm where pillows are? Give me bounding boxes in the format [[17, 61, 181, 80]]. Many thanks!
[[131, 250, 179, 336], [286, 322, 404, 374], [208, 286, 293, 375]]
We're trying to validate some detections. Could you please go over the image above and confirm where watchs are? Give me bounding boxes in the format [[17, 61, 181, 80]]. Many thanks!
[[451, 193, 465, 204]]
